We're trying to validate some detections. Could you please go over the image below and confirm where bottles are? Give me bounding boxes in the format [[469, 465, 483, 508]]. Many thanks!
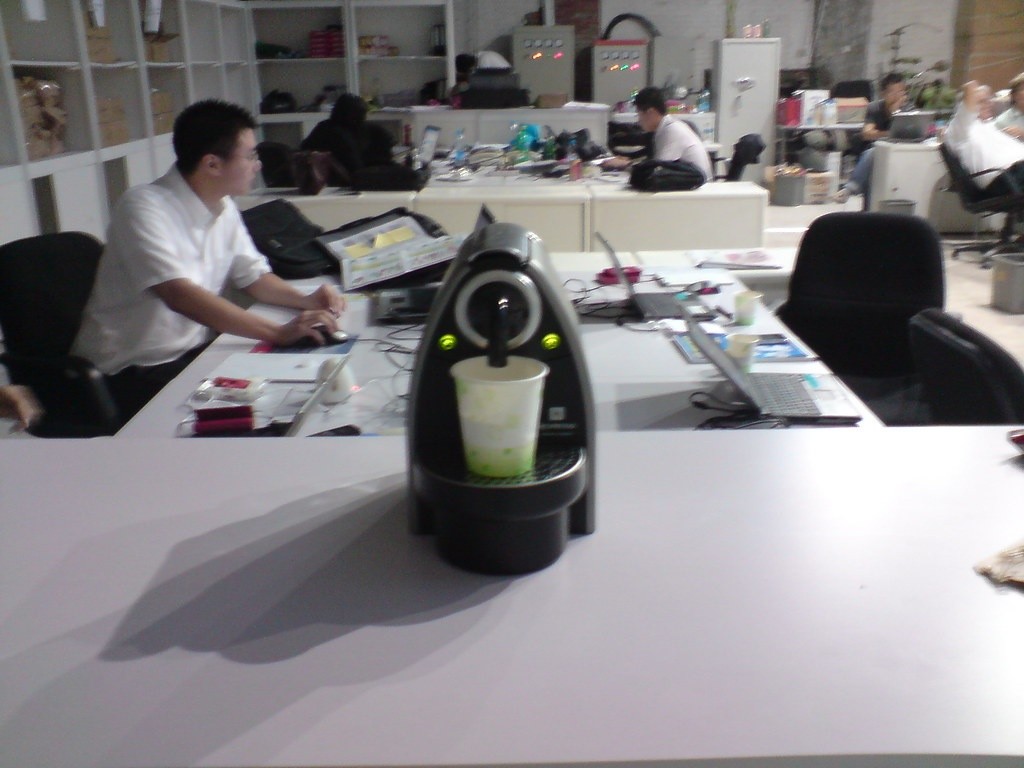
[[567, 138, 577, 164], [544, 133, 557, 159], [630, 87, 638, 101], [454, 130, 468, 168], [821, 100, 837, 125], [509, 119, 517, 150], [703, 89, 710, 112], [518, 126, 529, 164], [764, 19, 770, 38]]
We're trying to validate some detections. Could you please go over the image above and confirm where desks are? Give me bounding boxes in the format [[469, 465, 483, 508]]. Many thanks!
[[413, 180, 588, 252], [111, 273, 426, 435], [871, 140, 1008, 233], [0, 423, 1024, 768], [776, 123, 865, 165], [587, 181, 769, 251], [236, 187, 416, 230], [550, 250, 887, 432]]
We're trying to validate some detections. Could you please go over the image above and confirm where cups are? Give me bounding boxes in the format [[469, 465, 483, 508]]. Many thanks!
[[451, 357, 551, 478], [734, 291, 765, 326], [726, 334, 760, 374]]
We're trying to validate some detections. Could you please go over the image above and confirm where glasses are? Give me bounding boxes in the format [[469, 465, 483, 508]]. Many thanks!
[[222, 151, 259, 170]]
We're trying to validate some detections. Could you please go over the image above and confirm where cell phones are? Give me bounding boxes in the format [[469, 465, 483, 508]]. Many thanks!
[[758, 333, 788, 345]]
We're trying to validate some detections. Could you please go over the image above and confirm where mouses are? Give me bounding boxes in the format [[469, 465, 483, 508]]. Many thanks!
[[298, 323, 348, 346], [687, 280, 720, 295]]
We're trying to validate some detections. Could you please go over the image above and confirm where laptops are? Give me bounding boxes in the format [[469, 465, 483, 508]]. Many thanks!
[[882, 111, 936, 141], [595, 233, 717, 322], [376, 203, 497, 323], [677, 306, 863, 425], [410, 124, 443, 170]]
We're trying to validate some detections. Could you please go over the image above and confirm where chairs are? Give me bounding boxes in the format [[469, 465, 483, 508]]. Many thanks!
[[243, 199, 328, 278], [0, 230, 169, 439], [776, 212, 945, 403], [938, 144, 1024, 270], [911, 307, 1024, 428]]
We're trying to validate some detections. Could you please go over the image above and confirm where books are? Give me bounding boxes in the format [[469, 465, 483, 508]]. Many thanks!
[[665, 329, 815, 364]]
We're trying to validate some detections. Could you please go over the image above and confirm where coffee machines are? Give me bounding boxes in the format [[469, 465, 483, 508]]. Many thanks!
[[408, 222, 597, 577]]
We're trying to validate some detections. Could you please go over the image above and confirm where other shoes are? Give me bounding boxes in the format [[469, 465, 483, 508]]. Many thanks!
[[833, 191, 849, 204]]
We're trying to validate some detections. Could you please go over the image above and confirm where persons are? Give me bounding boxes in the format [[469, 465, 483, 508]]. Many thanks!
[[417, 51, 478, 106], [940, 72, 1024, 213], [600, 87, 713, 181], [65, 97, 346, 426], [299, 93, 424, 192], [864, 72, 920, 141]]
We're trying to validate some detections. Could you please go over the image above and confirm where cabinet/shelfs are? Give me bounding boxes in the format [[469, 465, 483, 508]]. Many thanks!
[[415, 109, 608, 150], [712, 37, 781, 184], [248, 0, 457, 125], [0, 0, 254, 187]]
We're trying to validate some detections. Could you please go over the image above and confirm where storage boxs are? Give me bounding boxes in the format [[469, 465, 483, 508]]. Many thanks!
[[96, 98, 128, 148], [150, 89, 177, 135], [769, 174, 804, 207], [310, 25, 345, 59], [832, 96, 869, 125], [15, 77, 68, 161], [778, 98, 800, 127], [357, 46, 400, 57], [358, 35, 390, 47], [800, 149, 841, 203], [792, 89, 830, 125], [84, 12, 115, 65], [140, 20, 179, 63]]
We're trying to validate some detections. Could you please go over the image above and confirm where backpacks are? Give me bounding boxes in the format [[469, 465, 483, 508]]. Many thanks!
[[628, 158, 704, 193], [241, 198, 340, 278]]
